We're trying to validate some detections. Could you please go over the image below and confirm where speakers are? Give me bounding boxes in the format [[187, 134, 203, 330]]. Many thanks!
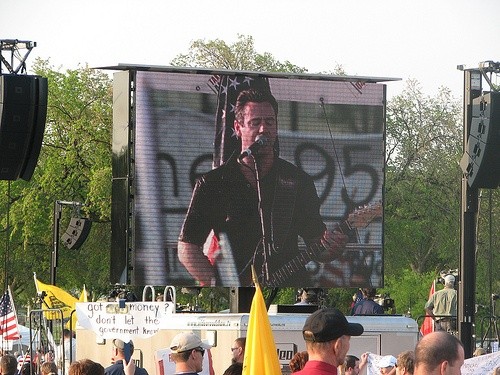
[[458, 92, 500, 190], [0, 73, 49, 181], [229, 287, 255, 314], [60, 216, 92, 250]]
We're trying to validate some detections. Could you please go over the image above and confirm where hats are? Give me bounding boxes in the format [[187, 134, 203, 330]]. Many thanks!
[[114, 338, 133, 350], [170, 330, 213, 353], [445, 275, 455, 282], [302, 307, 364, 342]]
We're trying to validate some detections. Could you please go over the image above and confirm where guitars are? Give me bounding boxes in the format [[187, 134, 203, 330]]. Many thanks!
[[249, 200, 381, 287]]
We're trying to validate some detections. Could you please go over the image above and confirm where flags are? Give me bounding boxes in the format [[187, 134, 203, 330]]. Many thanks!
[[418, 282, 434, 342], [242, 286, 282, 375], [0, 289, 22, 340], [35, 279, 79, 319], [198, 75, 280, 287], [66, 289, 87, 330]]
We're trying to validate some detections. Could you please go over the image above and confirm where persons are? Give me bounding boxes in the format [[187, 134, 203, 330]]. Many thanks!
[[294, 289, 317, 305], [104, 338, 149, 375], [425, 275, 458, 332], [68, 358, 135, 375], [223, 337, 246, 375], [169, 331, 212, 375], [352, 288, 384, 316], [291, 308, 364, 375], [0, 329, 76, 375], [177, 89, 348, 288], [414, 331, 464, 375], [473, 348, 500, 375], [289, 351, 414, 375]]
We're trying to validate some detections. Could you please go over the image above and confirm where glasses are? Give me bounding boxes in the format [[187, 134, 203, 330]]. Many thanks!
[[195, 348, 205, 356], [231, 347, 238, 351]]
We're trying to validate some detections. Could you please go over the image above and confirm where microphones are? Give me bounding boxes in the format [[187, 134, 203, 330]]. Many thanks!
[[239, 133, 269, 158]]
